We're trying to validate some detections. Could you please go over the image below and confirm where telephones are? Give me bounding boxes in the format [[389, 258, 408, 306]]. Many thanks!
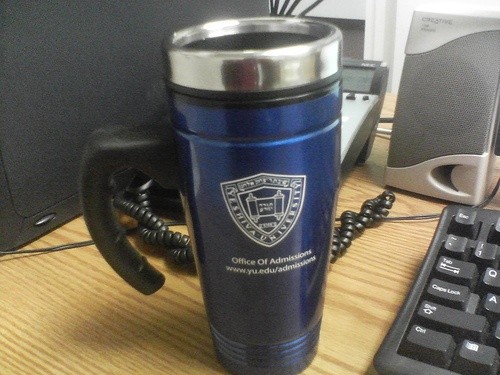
[[118, 58, 390, 218]]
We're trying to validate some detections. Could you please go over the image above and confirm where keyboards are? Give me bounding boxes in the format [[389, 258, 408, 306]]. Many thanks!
[[373, 204, 500, 375]]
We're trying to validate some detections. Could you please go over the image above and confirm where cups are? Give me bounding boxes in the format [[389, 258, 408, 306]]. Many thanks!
[[79, 16, 342, 375]]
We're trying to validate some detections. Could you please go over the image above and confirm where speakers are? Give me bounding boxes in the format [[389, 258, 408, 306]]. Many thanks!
[[385, 10, 500, 208]]
